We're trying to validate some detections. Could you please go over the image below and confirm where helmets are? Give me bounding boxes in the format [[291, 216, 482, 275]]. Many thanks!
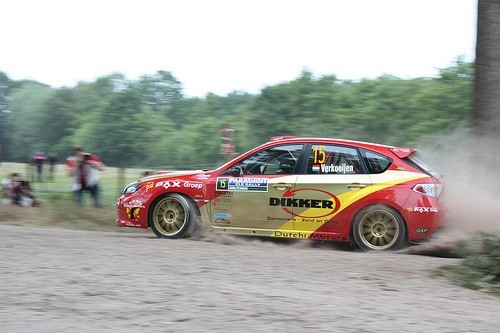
[[274, 154, 296, 173]]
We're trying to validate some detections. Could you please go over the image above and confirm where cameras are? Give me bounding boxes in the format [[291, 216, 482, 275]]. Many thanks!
[[82, 155, 88, 161]]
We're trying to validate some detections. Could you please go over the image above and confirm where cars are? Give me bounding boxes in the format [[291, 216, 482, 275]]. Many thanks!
[[116, 136, 447, 252]]
